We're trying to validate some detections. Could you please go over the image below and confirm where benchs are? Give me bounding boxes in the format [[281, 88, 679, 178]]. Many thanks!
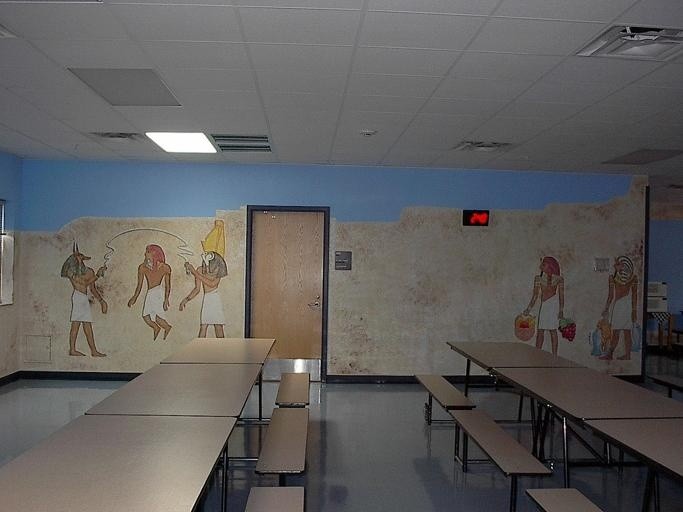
[[449, 409, 552, 512], [245, 486, 306, 512], [255, 408, 310, 485], [415, 375, 476, 462], [275, 372, 311, 406], [526, 488, 604, 512]]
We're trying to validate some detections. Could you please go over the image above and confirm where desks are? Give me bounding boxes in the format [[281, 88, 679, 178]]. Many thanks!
[[159, 338, 278, 460], [490, 365, 683, 463], [0, 415, 238, 512], [447, 342, 587, 424], [584, 419, 683, 512], [85, 363, 263, 461]]
